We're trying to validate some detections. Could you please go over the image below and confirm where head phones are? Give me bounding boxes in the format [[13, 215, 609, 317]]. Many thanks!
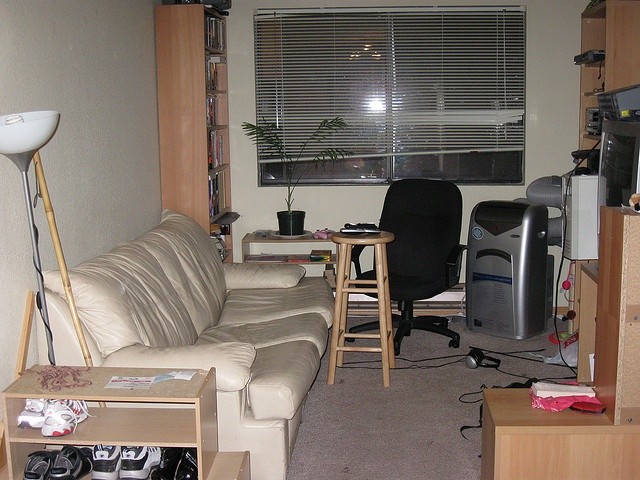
[[465, 348, 501, 369]]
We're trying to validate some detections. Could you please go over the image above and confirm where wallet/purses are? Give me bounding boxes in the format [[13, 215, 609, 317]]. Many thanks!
[[569, 402, 606, 415]]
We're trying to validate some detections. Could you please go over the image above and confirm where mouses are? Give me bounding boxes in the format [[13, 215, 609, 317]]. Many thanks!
[[575, 167, 591, 175]]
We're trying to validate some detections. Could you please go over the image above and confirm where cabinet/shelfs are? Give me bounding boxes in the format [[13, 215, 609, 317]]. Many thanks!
[[0, 363, 251, 480], [154, 2, 233, 262], [577, 0, 640, 168], [573, 260, 599, 383], [240, 231, 339, 292], [479, 205, 640, 480]]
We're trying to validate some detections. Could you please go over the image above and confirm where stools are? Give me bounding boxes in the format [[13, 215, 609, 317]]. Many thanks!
[[327, 229, 397, 388]]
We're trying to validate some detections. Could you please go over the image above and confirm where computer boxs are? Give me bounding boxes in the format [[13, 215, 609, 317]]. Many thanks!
[[559, 172, 600, 261]]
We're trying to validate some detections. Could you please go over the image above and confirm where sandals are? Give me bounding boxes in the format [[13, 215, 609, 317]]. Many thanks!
[[149, 447, 174, 480], [174, 448, 198, 480]]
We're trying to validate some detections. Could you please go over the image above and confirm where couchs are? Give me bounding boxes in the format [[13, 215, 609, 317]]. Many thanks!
[[40, 208, 335, 480]]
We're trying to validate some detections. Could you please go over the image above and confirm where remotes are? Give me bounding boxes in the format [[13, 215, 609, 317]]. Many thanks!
[[339, 227, 382, 234]]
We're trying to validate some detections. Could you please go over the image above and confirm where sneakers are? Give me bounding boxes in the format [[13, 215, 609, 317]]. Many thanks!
[[42, 399, 97, 437], [23, 449, 59, 480], [120, 446, 161, 480], [92, 444, 121, 480], [17, 398, 49, 429], [50, 444, 93, 480]]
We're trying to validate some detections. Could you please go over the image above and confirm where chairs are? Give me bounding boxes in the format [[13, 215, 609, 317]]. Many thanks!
[[345, 176, 469, 357]]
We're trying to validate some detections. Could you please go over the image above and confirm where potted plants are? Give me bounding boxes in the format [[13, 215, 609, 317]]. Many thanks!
[[241, 112, 356, 236]]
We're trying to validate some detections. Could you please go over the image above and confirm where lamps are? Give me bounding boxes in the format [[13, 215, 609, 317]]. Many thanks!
[[1, 110, 60, 366]]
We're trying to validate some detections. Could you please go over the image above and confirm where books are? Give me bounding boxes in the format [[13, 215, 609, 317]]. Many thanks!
[[532, 382, 596, 399], [204, 15, 226, 219]]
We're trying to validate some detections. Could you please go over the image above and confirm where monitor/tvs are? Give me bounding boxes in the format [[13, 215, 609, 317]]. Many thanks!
[[597, 121, 640, 208]]
